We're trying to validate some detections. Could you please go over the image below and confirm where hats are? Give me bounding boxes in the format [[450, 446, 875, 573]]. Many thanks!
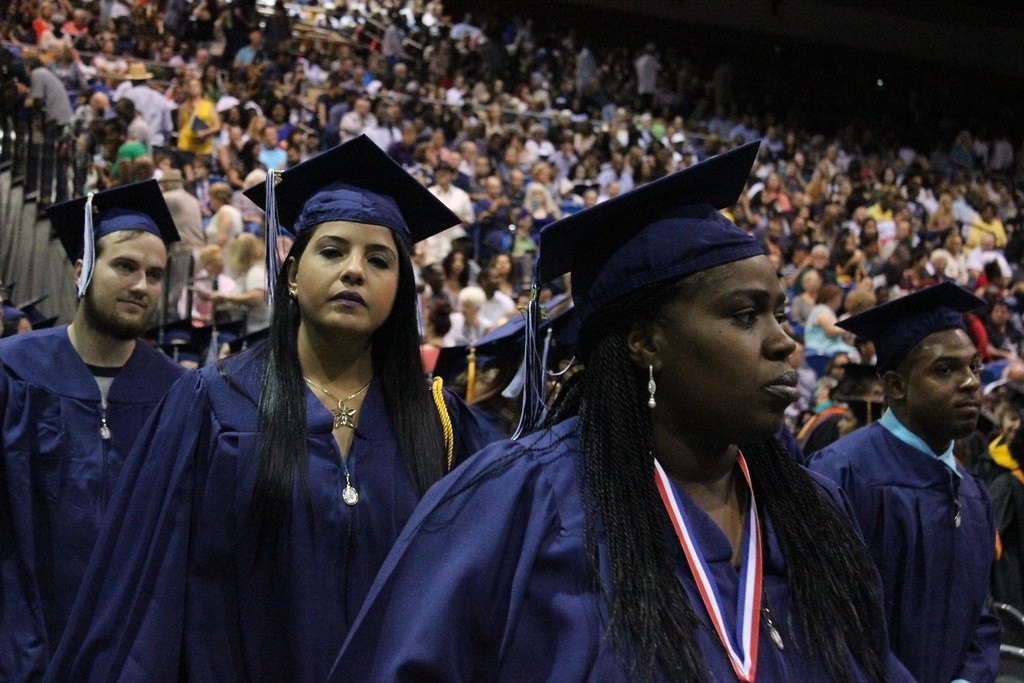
[[747, 182, 765, 200], [124, 62, 154, 80], [828, 364, 887, 425], [501, 138, 762, 439], [835, 280, 987, 375], [216, 94, 240, 113], [243, 134, 464, 304], [1, 292, 58, 334], [146, 307, 273, 363], [436, 293, 577, 443], [670, 134, 685, 142], [44, 178, 181, 299]]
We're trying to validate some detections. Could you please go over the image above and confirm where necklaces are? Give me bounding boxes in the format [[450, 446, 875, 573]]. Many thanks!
[[303, 376, 373, 427]]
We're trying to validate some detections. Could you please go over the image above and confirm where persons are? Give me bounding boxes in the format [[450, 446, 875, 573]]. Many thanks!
[[47, 135, 506, 683], [805, 281, 1001, 683], [0, 0, 1023, 608], [0, 179, 186, 683], [328, 143, 916, 683]]
